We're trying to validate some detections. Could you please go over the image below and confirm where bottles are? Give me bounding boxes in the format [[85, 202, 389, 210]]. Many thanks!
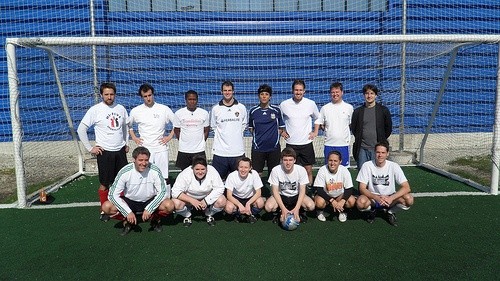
[[40, 190, 46, 202]]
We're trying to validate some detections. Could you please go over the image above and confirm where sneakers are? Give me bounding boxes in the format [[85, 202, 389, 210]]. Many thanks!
[[120, 222, 135, 236], [389, 213, 398, 226], [338, 212, 347, 222], [151, 217, 164, 233], [183, 218, 192, 227], [248, 214, 257, 224], [367, 210, 377, 223], [234, 213, 242, 223], [207, 216, 216, 226], [318, 210, 326, 222], [100, 210, 110, 222]]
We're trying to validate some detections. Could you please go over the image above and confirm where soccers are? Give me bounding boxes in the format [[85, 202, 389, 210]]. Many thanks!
[[282, 214, 300, 231]]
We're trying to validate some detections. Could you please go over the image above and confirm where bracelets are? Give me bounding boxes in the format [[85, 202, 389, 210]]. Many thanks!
[[331, 199, 335, 203]]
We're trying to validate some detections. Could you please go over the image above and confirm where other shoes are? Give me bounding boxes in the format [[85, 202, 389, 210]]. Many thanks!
[[299, 213, 308, 221], [272, 212, 282, 224]]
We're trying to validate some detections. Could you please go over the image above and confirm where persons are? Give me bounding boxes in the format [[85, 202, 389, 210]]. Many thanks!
[[350, 85, 393, 172], [171, 155, 226, 228], [208, 81, 250, 186], [77, 82, 130, 224], [223, 158, 269, 225], [354, 142, 414, 228], [318, 82, 356, 173], [265, 147, 315, 225], [173, 89, 210, 175], [280, 80, 320, 191], [128, 84, 176, 186], [108, 147, 175, 237], [311, 150, 358, 222], [248, 84, 286, 180]]
